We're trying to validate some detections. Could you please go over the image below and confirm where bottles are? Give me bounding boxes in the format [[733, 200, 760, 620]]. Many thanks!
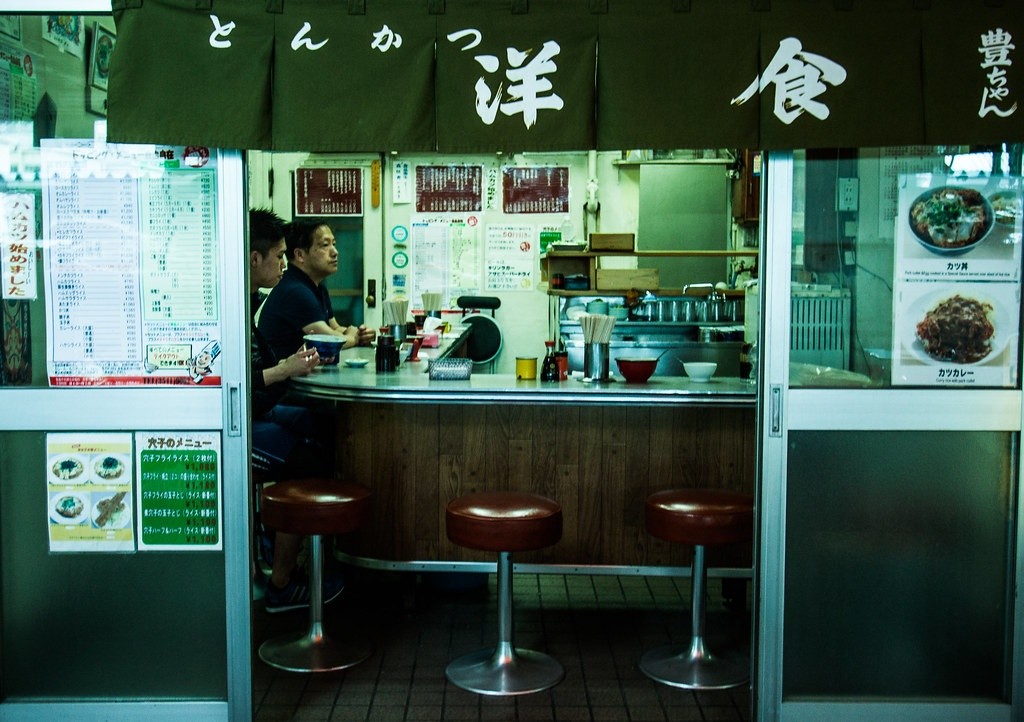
[[554, 351, 568, 382], [559, 215, 575, 241], [551, 273, 566, 288], [378, 327, 390, 336], [540, 341, 560, 382]]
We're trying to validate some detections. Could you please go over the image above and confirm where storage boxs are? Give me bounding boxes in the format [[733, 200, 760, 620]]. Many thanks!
[[594, 268, 659, 291], [424, 310, 441, 319], [428, 358, 473, 380], [589, 232, 635, 253], [417, 330, 442, 348], [386, 323, 407, 342], [575, 343, 616, 384]]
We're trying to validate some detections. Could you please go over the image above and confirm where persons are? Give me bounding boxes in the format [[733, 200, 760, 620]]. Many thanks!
[[256, 218, 377, 407], [249, 207, 346, 615]]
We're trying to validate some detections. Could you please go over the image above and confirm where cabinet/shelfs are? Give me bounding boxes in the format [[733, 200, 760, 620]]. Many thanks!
[[543, 251, 758, 298]]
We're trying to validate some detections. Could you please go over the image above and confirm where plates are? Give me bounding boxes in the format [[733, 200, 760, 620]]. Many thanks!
[[552, 241, 588, 252], [49, 451, 131, 531], [344, 358, 369, 368]]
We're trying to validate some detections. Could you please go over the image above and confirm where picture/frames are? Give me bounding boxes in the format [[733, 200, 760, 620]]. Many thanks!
[[87, 21, 118, 90]]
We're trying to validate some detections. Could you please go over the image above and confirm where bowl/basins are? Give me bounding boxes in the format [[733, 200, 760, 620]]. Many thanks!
[[614, 356, 659, 383], [561, 297, 628, 323], [303, 333, 348, 359], [906, 184, 996, 258], [684, 362, 717, 384], [985, 190, 1024, 232], [406, 334, 424, 362], [896, 289, 1013, 367]]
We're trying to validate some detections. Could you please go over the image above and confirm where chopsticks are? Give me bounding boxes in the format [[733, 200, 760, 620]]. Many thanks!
[[302, 344, 313, 363], [579, 313, 616, 343]]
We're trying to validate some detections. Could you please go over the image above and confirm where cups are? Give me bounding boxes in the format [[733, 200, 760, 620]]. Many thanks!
[[738, 354, 757, 385], [379, 335, 394, 349], [515, 356, 538, 381], [643, 299, 741, 323], [374, 345, 398, 374]]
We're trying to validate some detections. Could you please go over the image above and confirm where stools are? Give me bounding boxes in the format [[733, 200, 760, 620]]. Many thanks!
[[446, 491, 564, 695], [641, 486, 748, 690], [260, 479, 376, 677]]
